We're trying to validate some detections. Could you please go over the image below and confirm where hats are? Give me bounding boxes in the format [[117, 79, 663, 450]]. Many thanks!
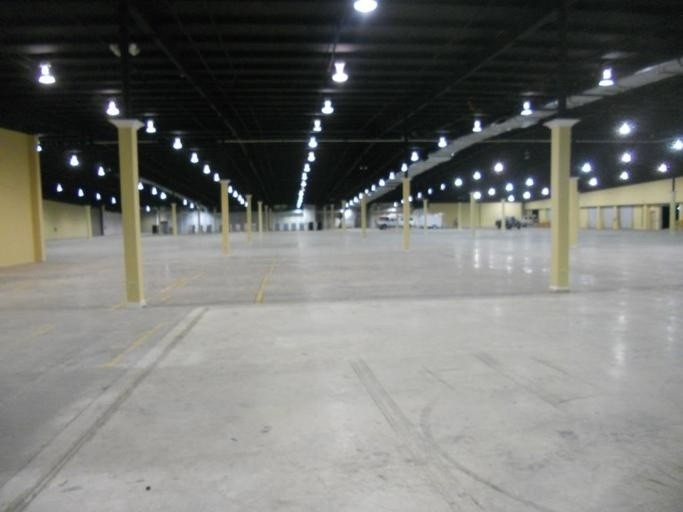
[[494, 216, 522, 230], [519, 214, 538, 226]]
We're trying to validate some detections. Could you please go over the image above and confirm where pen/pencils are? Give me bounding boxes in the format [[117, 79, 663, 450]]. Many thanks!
[[409, 212, 441, 230], [374, 211, 416, 231]]
